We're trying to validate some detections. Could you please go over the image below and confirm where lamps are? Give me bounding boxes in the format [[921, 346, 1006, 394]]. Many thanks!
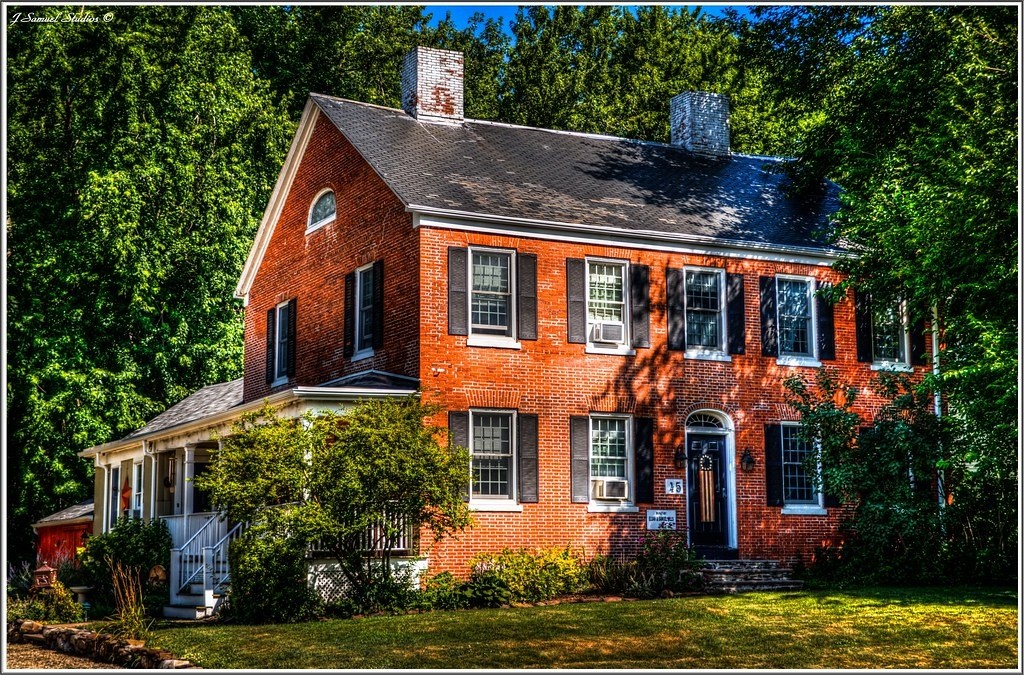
[[740, 447, 756, 473], [673, 446, 689, 470], [32, 560, 57, 587]]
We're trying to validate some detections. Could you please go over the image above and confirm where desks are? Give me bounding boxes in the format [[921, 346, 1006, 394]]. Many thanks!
[[71, 586, 93, 604]]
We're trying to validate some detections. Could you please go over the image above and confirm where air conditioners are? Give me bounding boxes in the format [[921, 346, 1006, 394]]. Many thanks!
[[593, 321, 625, 344], [595, 480, 629, 500]]
